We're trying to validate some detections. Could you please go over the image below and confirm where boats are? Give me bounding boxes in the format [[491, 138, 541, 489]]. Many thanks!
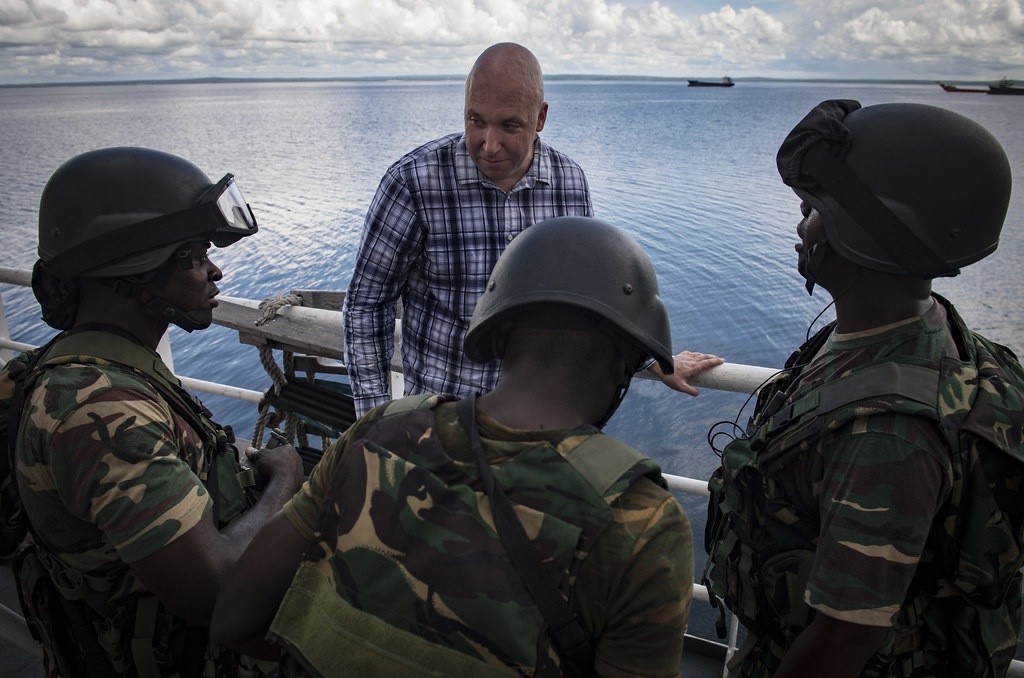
[[937, 80, 987, 93], [685, 78, 735, 90], [988, 82, 1024, 96]]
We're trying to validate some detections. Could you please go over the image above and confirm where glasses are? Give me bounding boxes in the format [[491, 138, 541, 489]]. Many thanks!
[[201, 173, 257, 249]]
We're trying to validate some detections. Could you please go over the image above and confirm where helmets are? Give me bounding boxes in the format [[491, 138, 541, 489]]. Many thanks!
[[778, 99, 1013, 279], [463, 216, 675, 376], [40, 146, 259, 277]]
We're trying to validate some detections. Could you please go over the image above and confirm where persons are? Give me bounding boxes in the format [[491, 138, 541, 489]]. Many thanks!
[[210, 217, 695, 678], [345, 43, 725, 420], [0, 148, 303, 678], [701, 100, 1024, 678]]
